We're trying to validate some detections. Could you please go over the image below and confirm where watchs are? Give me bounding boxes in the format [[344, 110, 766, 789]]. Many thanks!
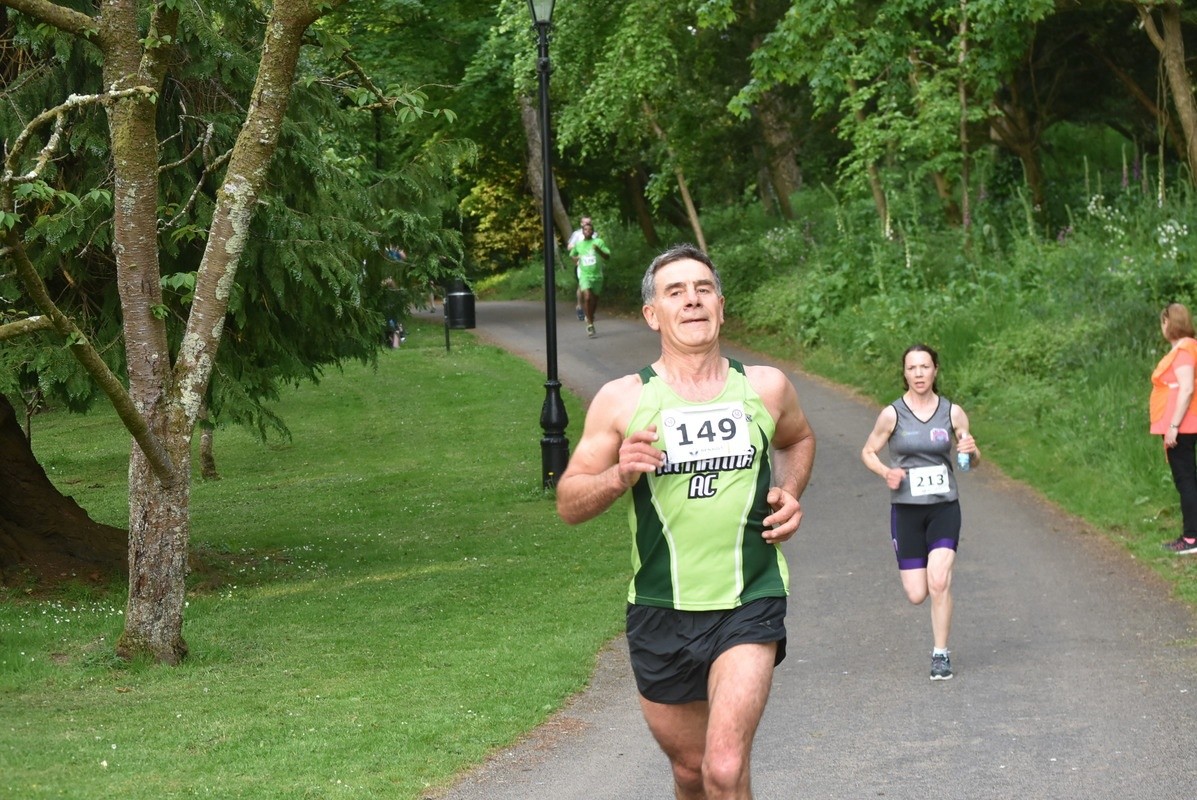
[[1169, 424, 1179, 428]]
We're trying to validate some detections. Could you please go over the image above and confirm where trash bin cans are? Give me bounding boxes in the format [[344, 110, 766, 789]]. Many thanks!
[[446, 277, 476, 329]]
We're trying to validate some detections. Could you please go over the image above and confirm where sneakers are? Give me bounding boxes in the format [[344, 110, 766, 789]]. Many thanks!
[[576, 306, 584, 322], [1168, 537, 1197, 554], [930, 653, 953, 682], [585, 325, 595, 338]]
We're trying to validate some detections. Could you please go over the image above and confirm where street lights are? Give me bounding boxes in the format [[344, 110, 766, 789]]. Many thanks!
[[528, 0, 568, 493]]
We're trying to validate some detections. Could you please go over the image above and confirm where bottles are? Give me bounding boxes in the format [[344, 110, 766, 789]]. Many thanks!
[[956, 432, 970, 473]]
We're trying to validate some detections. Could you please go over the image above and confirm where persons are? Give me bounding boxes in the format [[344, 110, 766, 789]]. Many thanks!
[[556, 247, 816, 800], [363, 242, 407, 349], [860, 344, 981, 681], [1149, 303, 1197, 555], [567, 216, 610, 337]]
